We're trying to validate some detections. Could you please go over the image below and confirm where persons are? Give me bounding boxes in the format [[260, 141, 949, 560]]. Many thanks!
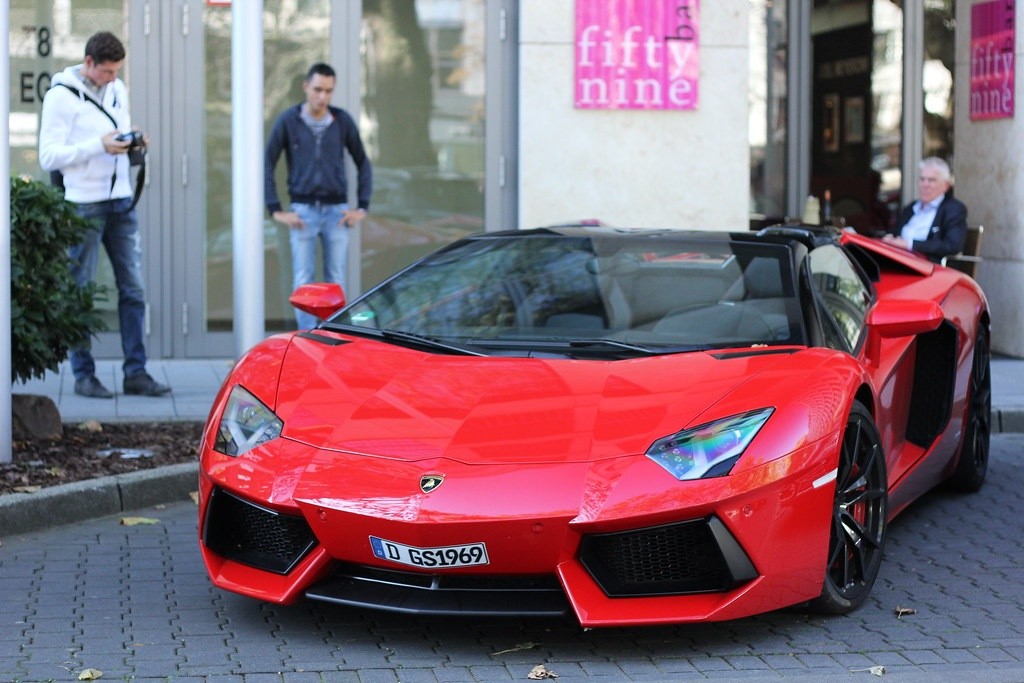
[[38, 30, 173, 401], [843, 157, 969, 258], [264, 63, 372, 331]]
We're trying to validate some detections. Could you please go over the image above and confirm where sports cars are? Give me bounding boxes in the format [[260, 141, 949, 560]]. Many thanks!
[[198, 221, 992, 629]]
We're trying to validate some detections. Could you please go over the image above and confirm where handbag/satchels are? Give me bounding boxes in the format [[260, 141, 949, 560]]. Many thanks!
[[51, 171, 65, 196]]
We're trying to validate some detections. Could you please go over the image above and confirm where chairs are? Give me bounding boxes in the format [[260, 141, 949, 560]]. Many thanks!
[[719, 243, 810, 341], [511, 252, 632, 334], [942, 225, 986, 279]]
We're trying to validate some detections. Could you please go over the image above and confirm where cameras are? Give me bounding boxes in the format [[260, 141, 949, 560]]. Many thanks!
[[116, 131, 144, 167]]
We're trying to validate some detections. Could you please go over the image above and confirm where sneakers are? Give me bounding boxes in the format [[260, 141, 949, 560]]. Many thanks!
[[75, 374, 113, 398], [124, 374, 171, 395]]
